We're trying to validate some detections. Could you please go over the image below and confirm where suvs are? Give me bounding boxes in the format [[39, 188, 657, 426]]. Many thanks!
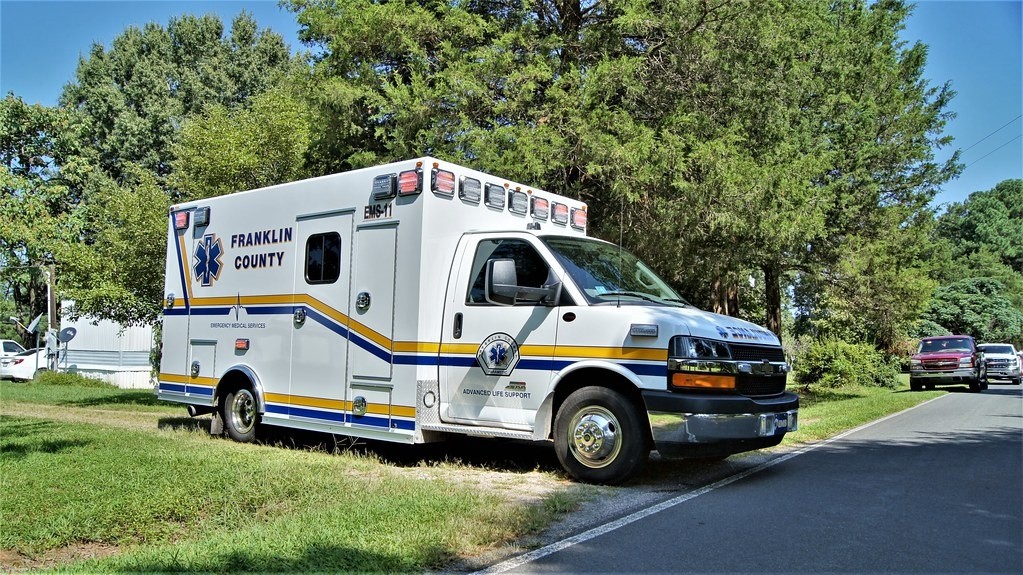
[[909, 335, 988, 392], [976, 343, 1023, 385]]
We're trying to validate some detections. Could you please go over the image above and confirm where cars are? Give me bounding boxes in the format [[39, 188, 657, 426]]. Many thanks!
[[0, 347, 49, 382], [0, 339, 27, 357]]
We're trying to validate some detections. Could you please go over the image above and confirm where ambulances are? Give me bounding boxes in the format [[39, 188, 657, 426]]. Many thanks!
[[156, 154, 801, 487]]
